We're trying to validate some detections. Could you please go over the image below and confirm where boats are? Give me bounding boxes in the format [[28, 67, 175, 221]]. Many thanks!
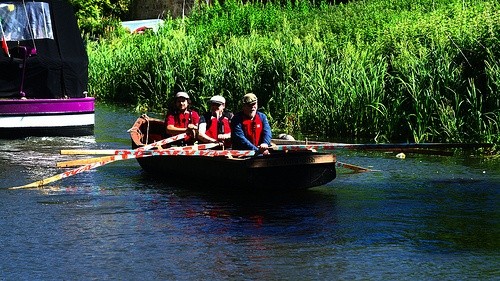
[[130, 114, 337, 190], [0, 92, 96, 136]]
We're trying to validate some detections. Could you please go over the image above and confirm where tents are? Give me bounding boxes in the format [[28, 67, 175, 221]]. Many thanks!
[[0, 0, 88, 99]]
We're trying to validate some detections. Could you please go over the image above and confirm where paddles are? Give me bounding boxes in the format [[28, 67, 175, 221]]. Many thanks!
[[270, 137, 495, 175], [14, 134, 256, 191]]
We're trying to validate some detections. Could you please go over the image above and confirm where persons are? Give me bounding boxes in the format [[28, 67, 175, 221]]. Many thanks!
[[197, 95, 233, 148], [231, 93, 272, 157], [165, 92, 200, 145]]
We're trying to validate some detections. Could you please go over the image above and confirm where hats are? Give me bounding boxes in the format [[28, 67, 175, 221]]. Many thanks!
[[242, 93, 257, 104], [175, 92, 189, 98], [210, 95, 226, 105]]
